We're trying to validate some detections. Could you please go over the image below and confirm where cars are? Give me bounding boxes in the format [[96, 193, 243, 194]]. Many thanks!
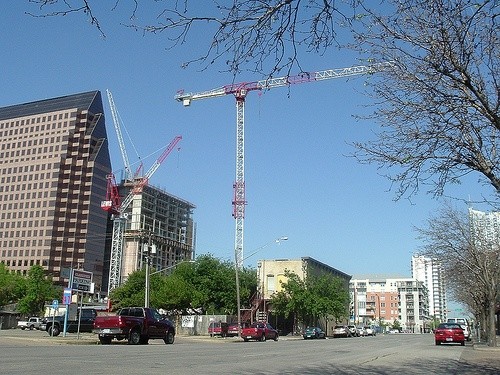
[[400, 329, 412, 333], [304, 327, 326, 339], [208, 320, 244, 337], [333, 325, 377, 338], [377, 326, 399, 335], [432, 318, 476, 345]]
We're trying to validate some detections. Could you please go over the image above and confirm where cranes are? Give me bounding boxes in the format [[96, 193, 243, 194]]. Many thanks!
[[100, 89, 185, 302], [173, 59, 402, 271]]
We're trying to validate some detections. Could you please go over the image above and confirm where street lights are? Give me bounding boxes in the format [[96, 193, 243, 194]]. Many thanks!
[[235, 235, 288, 342], [145, 260, 195, 309]]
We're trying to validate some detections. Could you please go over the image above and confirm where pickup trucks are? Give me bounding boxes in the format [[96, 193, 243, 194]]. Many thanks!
[[18, 318, 42, 331], [240, 322, 279, 343], [93, 307, 176, 344], [40, 308, 97, 336]]
